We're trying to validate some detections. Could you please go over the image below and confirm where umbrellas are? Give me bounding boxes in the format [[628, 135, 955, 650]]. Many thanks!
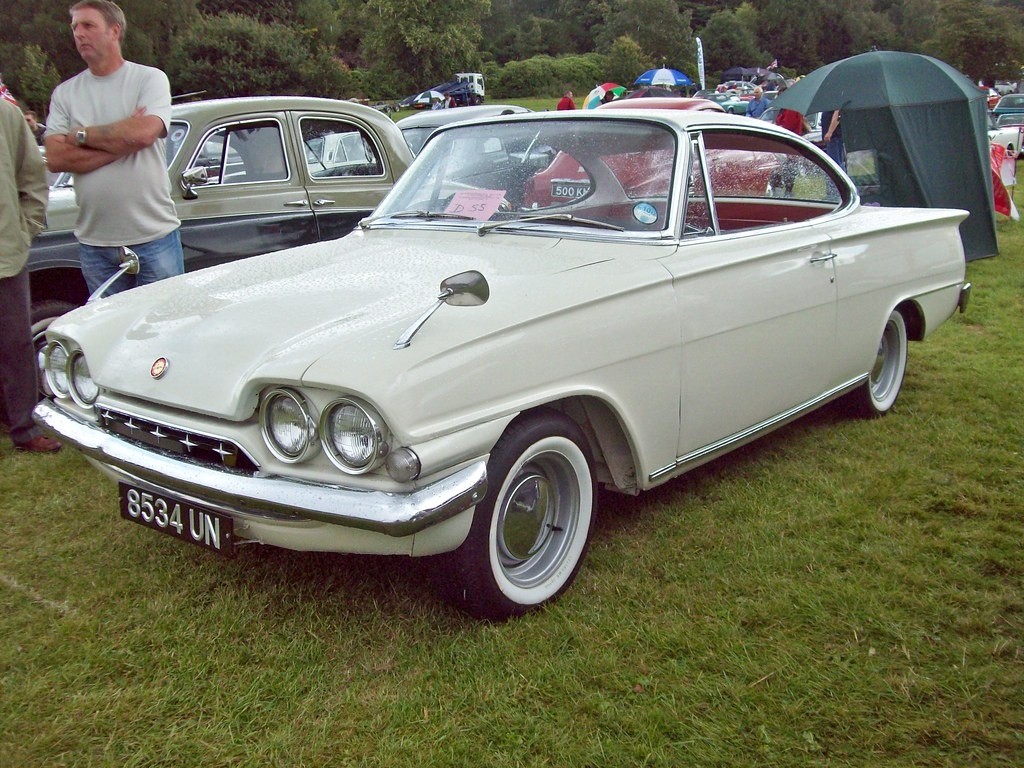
[[581, 62, 693, 110], [414, 90, 445, 104], [722, 66, 790, 90]]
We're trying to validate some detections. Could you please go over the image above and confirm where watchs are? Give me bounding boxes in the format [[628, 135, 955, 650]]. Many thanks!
[[75, 126, 88, 144]]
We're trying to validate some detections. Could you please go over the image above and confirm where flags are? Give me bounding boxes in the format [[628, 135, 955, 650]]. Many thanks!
[[767, 59, 777, 70], [0, 73, 19, 106]]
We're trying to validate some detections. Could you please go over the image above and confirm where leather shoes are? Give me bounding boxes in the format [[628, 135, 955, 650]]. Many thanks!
[[12, 434, 63, 453]]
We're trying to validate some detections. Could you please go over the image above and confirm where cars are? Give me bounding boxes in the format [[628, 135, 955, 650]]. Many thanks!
[[981, 86, 1024, 153], [195, 105, 372, 189], [23, 97, 509, 402], [392, 104, 565, 216], [523, 96, 786, 212], [692, 81, 782, 115], [30, 101, 969, 620]]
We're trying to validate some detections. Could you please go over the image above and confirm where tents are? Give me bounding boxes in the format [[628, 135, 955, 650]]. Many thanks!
[[766, 44, 1000, 263]]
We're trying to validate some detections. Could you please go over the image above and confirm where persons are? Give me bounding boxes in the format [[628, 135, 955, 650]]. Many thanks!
[[0, 97, 62, 453], [597, 91, 615, 107], [718, 80, 775, 94], [25, 110, 46, 146], [674, 83, 700, 98], [557, 90, 576, 110], [821, 110, 843, 201], [43, 0, 187, 299], [745, 86, 770, 118], [766, 109, 812, 196], [432, 92, 456, 110]]
